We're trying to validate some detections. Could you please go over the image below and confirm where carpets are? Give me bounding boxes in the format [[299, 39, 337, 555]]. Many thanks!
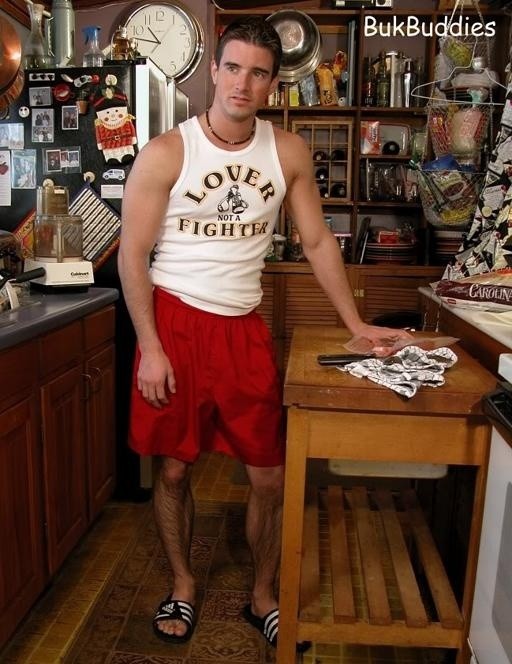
[[59, 494, 304, 663]]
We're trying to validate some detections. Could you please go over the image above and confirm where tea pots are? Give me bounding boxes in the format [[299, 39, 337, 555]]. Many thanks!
[[9, 56, 190, 495]]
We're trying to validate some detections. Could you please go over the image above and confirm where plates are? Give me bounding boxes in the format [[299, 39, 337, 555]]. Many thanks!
[[431, 228, 469, 256], [355, 216, 418, 265]]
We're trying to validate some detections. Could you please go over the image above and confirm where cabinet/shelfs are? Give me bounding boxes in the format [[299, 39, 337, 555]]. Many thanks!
[[0, 339, 48, 655], [204, 0, 512, 369], [37, 305, 117, 585]]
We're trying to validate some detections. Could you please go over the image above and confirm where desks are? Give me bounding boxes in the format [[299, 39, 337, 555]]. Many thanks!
[[275, 324, 501, 663]]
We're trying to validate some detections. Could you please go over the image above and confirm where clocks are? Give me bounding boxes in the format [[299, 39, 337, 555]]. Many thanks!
[[120, 1, 204, 85]]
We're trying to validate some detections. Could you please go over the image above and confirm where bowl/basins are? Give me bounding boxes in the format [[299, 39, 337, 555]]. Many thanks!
[[438, 85, 494, 110], [262, 10, 324, 84]]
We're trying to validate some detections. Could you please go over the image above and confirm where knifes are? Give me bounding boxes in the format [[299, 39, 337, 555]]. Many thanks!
[[316, 350, 453, 365]]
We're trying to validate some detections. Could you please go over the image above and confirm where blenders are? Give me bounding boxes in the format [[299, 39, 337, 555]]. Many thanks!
[[23, 184, 96, 289]]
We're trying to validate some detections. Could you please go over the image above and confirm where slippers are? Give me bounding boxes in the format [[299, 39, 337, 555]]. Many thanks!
[[244, 603, 311, 653], [153, 592, 196, 644]]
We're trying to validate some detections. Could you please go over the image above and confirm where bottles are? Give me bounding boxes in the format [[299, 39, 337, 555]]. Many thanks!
[[363, 47, 424, 107], [312, 148, 348, 199], [268, 233, 288, 261], [49, 0, 77, 67], [109, 26, 130, 60], [21, 3, 52, 70]]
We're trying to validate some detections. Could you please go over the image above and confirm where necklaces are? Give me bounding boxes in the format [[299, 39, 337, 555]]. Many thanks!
[[205, 103, 256, 145]]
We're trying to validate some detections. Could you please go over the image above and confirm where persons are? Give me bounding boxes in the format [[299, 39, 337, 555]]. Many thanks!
[[48, 153, 78, 170], [117, 16, 417, 651], [33, 91, 52, 142]]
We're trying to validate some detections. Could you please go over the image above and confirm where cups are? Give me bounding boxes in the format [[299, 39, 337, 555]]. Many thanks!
[[129, 37, 138, 55]]
